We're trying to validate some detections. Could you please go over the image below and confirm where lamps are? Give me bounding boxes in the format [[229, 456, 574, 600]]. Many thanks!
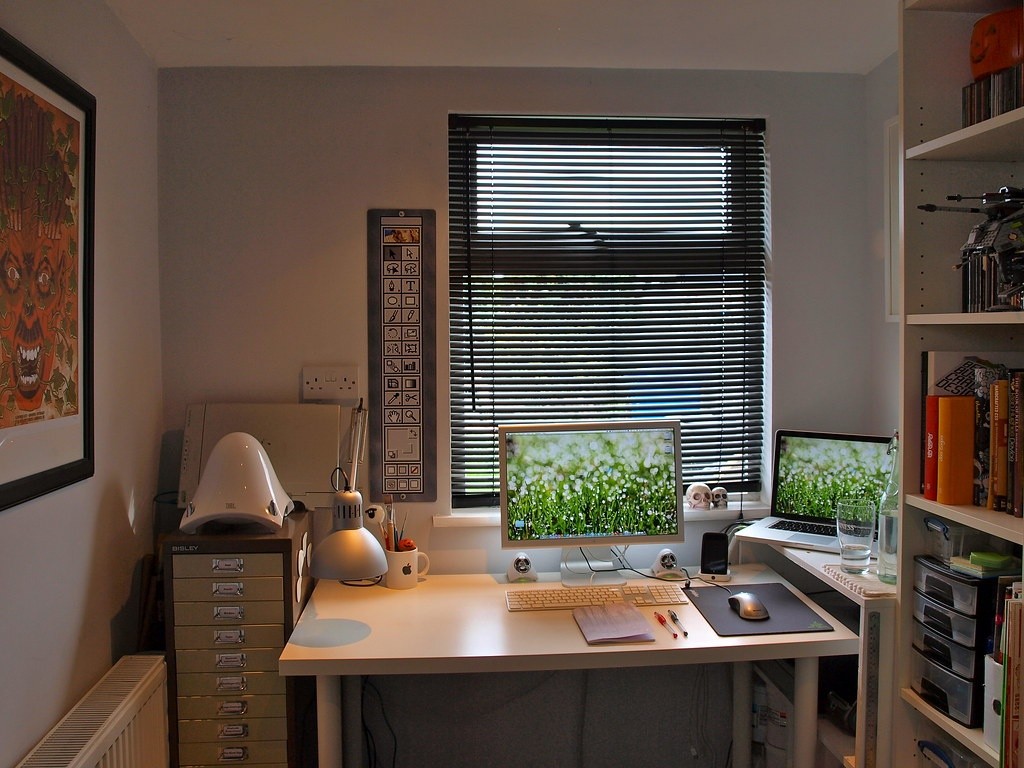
[[304, 395, 388, 582]]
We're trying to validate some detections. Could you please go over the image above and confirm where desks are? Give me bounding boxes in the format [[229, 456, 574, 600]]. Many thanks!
[[279, 562, 861, 768]]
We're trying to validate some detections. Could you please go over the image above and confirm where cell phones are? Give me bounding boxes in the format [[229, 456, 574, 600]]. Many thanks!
[[700, 532, 728, 575]]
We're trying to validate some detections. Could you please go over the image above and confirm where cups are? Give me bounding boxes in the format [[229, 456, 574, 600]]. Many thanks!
[[836, 499, 875, 575], [385, 546, 429, 590]]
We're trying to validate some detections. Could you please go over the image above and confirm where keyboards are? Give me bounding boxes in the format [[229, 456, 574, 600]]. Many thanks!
[[505, 583, 689, 613]]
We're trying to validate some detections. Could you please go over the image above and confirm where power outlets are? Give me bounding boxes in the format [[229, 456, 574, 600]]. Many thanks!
[[302, 366, 360, 401]]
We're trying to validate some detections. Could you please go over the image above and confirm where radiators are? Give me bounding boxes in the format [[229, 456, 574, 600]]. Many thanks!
[[19, 652, 171, 768]]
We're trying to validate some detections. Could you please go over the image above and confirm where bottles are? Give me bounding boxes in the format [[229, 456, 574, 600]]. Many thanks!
[[877, 429, 898, 585]]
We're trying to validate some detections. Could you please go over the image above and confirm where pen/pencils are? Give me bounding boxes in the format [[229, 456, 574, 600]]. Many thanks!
[[654, 612, 678, 638], [385, 533, 389, 551], [387, 523, 394, 551], [667, 610, 688, 636]]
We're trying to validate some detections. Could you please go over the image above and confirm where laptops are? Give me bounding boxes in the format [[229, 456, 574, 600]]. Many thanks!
[[734, 428, 894, 559]]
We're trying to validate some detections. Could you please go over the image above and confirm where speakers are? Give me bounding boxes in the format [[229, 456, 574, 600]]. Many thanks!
[[651, 548, 687, 583], [507, 552, 537, 581]]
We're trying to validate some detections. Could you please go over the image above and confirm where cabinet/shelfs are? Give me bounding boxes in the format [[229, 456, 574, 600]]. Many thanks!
[[746, 543, 896, 768], [886, 0, 1024, 768], [158, 509, 315, 768]]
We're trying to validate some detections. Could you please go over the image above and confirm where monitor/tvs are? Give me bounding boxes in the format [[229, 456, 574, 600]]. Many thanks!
[[497, 419, 685, 587]]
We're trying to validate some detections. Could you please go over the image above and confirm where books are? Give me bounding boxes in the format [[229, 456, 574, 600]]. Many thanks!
[[919, 350, 1024, 518], [993, 575, 1022, 768], [961, 251, 1024, 312], [961, 63, 1024, 128]]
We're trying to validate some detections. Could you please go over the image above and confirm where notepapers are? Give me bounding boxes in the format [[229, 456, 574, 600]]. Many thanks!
[[949, 556, 1016, 580], [969, 552, 1011, 568]]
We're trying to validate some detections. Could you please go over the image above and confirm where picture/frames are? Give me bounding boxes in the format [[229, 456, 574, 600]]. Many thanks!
[[0, 26, 99, 511]]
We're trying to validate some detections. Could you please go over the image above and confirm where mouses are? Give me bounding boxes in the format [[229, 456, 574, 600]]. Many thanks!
[[727, 591, 770, 621]]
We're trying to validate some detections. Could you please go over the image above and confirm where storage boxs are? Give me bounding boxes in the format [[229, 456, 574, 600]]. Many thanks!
[[909, 553, 997, 614], [909, 646, 984, 729], [982, 652, 1004, 754], [911, 617, 985, 680], [912, 587, 993, 649], [918, 514, 979, 565]]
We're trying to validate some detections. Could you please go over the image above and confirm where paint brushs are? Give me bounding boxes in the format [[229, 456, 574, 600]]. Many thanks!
[[385, 501, 408, 552]]
